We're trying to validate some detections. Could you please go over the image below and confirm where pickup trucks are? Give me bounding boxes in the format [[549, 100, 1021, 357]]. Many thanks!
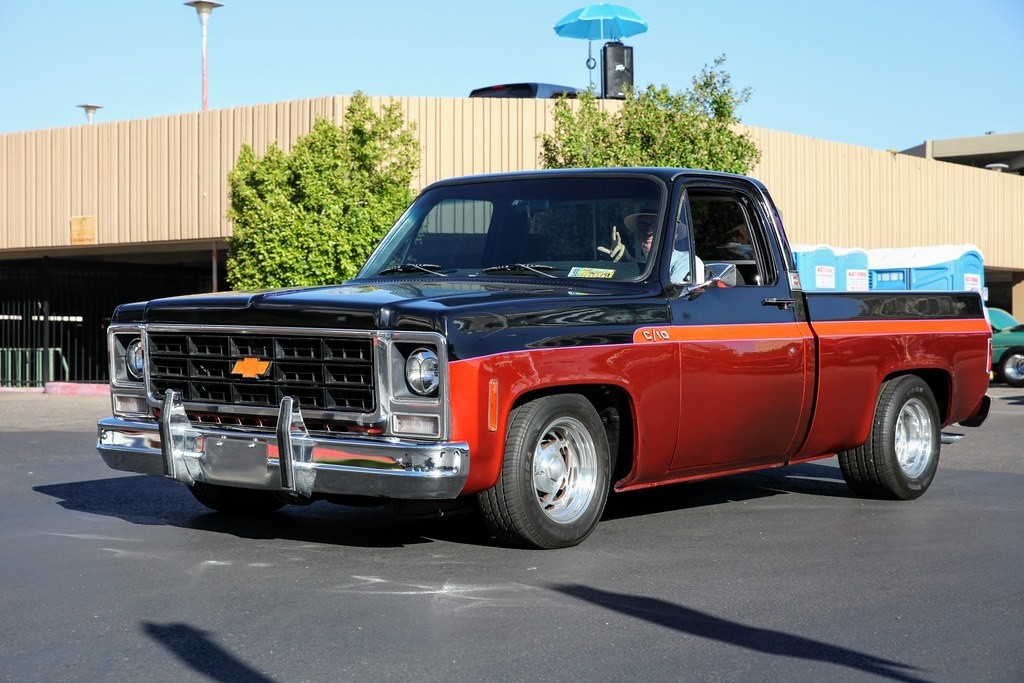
[[97, 167, 995, 549]]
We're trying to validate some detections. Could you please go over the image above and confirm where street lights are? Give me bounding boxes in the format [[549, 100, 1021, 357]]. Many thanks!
[[184, 0, 223, 111], [79, 103, 103, 126]]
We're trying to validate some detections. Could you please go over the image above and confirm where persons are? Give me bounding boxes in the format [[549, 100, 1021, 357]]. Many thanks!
[[596, 192, 704, 285]]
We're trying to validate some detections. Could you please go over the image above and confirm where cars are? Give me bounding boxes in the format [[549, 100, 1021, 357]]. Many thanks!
[[985, 308, 1024, 388]]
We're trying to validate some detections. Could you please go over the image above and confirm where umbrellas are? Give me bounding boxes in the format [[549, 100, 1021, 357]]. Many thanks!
[[553, 4, 648, 47]]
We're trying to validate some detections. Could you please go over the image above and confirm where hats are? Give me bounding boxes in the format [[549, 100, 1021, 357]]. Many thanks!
[[623, 201, 687, 241], [724, 213, 746, 236]]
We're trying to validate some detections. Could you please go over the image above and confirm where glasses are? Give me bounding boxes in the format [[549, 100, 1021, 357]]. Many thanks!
[[638, 222, 657, 232]]
